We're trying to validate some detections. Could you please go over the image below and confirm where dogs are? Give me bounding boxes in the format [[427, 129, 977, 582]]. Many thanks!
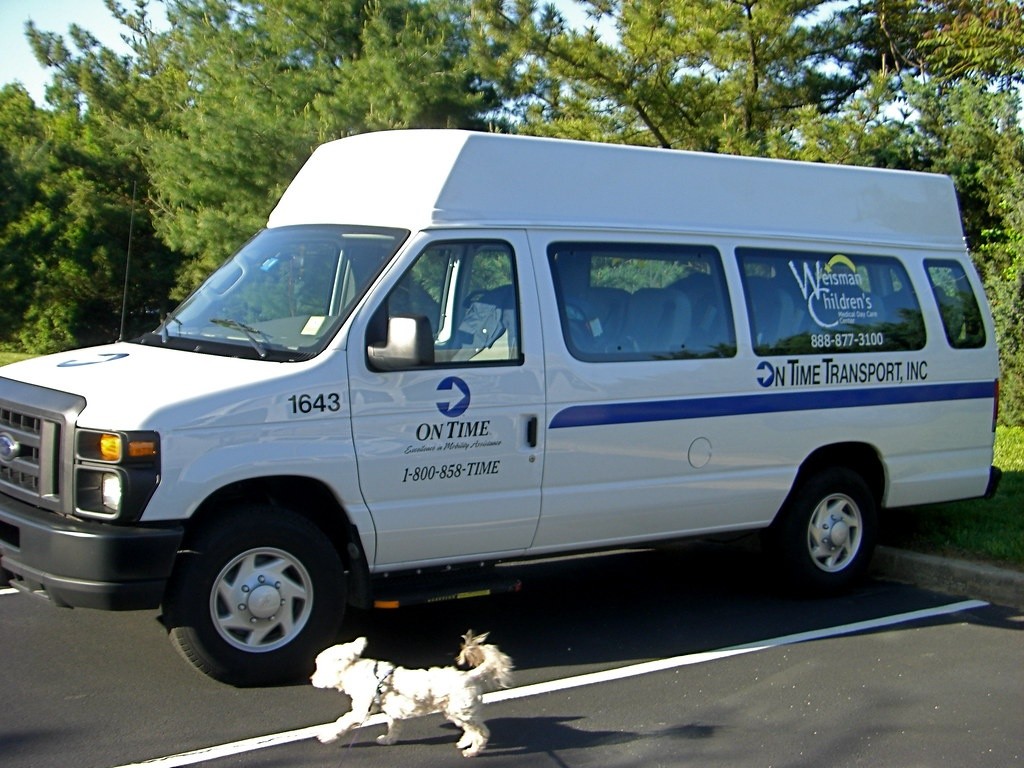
[[307, 631, 517, 759]]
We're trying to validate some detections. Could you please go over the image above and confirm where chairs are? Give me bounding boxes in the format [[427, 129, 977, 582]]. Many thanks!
[[664, 272, 794, 347], [577, 287, 629, 340], [800, 283, 966, 339], [451, 283, 518, 349], [616, 287, 691, 354]]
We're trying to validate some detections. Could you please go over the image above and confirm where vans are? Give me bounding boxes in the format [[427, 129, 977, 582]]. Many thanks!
[[0, 125, 1005, 693]]
